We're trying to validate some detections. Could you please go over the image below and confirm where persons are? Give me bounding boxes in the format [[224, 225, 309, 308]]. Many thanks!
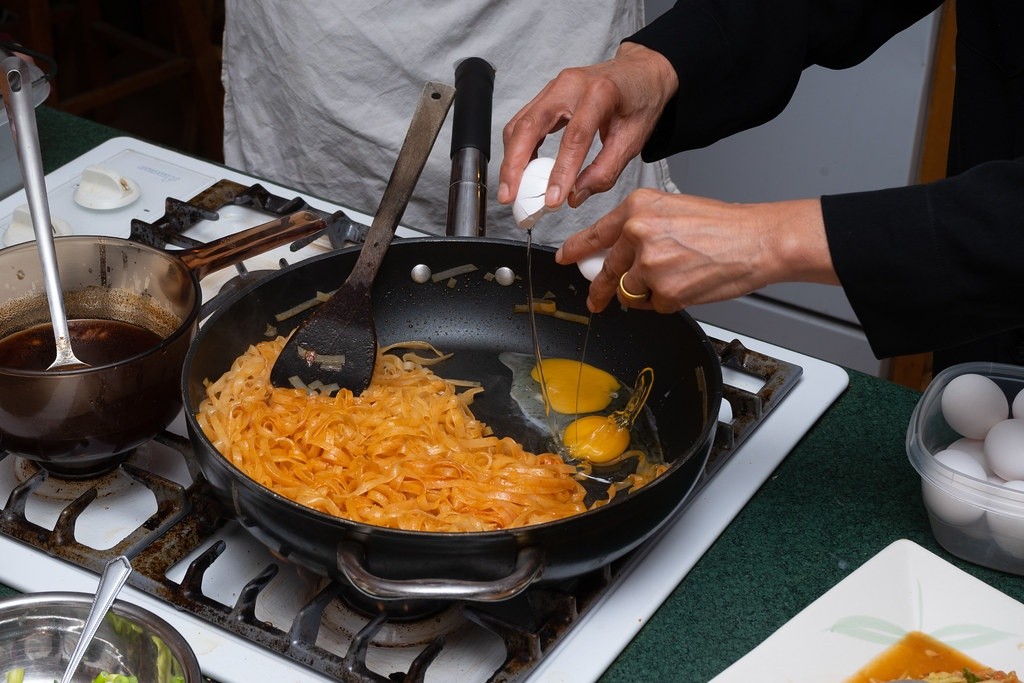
[[219, 0, 682, 290], [496, 0, 1024, 362]]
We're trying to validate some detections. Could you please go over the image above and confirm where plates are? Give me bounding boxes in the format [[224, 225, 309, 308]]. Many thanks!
[[708, 537, 1024, 683]]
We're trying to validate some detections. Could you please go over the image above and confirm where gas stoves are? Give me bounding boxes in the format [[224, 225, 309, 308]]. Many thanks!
[[2, 131, 850, 682]]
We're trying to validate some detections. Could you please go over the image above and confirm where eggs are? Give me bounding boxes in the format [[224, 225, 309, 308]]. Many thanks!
[[920, 372, 1024, 563], [497, 155, 654, 475]]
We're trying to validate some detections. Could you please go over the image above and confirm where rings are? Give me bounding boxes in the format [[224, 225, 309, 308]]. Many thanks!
[[619, 271, 648, 301]]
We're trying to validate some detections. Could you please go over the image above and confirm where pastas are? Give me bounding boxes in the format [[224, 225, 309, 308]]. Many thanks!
[[193, 319, 670, 535]]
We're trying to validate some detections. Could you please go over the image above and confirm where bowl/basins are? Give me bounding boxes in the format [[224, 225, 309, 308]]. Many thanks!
[[903, 362, 1024, 579], [2, 590, 197, 683]]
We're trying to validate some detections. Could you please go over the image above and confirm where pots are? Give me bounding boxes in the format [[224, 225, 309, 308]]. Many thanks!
[[177, 55, 724, 601], [1, 211, 351, 460]]
